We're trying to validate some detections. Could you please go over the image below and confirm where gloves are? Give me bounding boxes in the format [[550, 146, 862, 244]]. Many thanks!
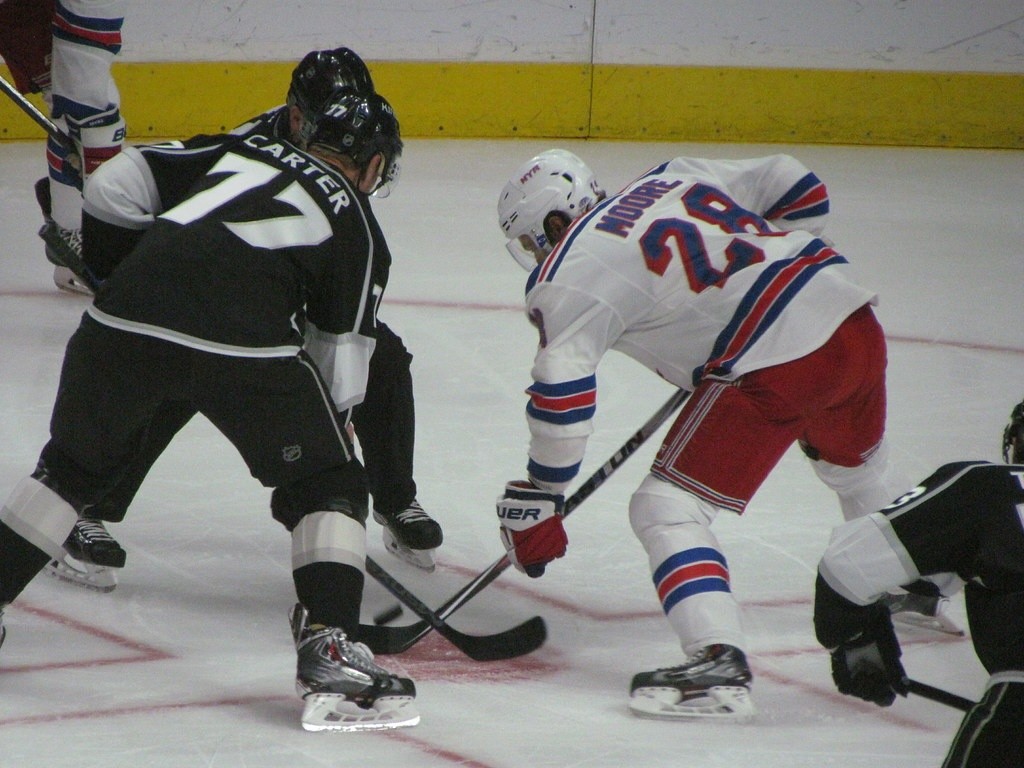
[[814, 603, 910, 707], [495, 479, 571, 578]]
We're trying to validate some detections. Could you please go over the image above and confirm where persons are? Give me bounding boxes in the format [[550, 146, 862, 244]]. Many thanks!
[[0, 2, 444, 727], [497, 148, 962, 717], [815, 399, 1024, 768]]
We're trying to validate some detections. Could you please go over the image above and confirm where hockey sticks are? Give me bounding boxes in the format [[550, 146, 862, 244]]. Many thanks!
[[1, 77, 84, 175], [898, 677, 977, 714], [352, 388, 692, 655], [38, 223, 546, 660]]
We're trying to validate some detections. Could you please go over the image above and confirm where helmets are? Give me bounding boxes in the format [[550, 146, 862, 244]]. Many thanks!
[[301, 88, 404, 185], [286, 47, 374, 123], [496, 149, 606, 272], [1003, 401, 1024, 464]]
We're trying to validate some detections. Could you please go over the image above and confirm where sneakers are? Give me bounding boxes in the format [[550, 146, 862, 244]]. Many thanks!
[[288, 603, 421, 732], [628, 643, 757, 726], [372, 497, 443, 572], [928, 596, 969, 637], [34, 177, 95, 295], [42, 516, 126, 593]]
